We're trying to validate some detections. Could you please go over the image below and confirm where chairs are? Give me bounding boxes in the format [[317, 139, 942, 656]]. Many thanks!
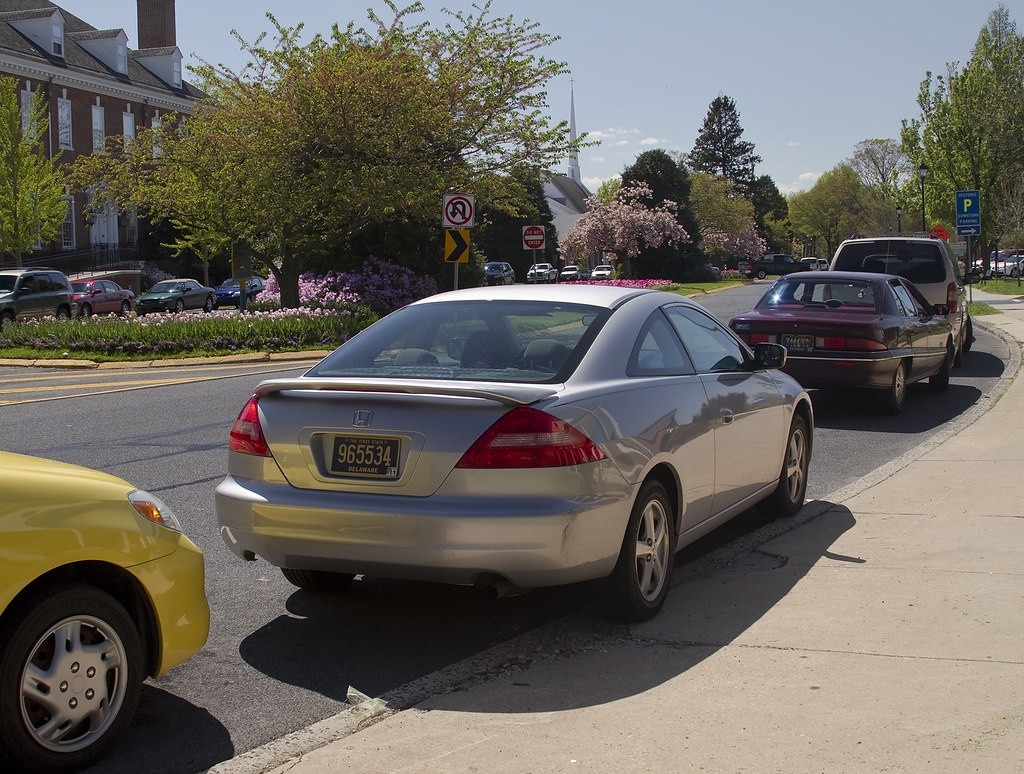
[[461, 330, 515, 369], [918, 262, 938, 278], [524, 339, 571, 370], [865, 260, 885, 273], [394, 349, 439, 367]]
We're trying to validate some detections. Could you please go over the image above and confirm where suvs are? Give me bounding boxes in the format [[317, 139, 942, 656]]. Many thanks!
[[560, 266, 582, 281], [827, 231, 972, 368], [0, 267, 75, 332]]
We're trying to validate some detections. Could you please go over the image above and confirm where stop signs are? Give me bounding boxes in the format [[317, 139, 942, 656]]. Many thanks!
[[522, 226, 546, 250]]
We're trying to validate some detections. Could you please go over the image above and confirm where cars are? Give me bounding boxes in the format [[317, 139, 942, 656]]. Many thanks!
[[214, 284, 816, 619], [801, 258, 829, 271], [957, 248, 1024, 278], [67, 279, 136, 319], [0, 449, 208, 772], [591, 265, 615, 280], [482, 262, 515, 285], [727, 270, 953, 419], [581, 269, 592, 280], [215, 275, 266, 309], [136, 278, 216, 313]]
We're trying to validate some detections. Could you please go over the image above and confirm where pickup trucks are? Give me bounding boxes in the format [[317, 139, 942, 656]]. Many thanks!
[[526, 263, 558, 284], [737, 254, 810, 279]]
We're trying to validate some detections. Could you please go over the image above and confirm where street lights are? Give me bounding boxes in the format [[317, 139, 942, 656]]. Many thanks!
[[896, 206, 902, 232], [918, 163, 928, 232]]
